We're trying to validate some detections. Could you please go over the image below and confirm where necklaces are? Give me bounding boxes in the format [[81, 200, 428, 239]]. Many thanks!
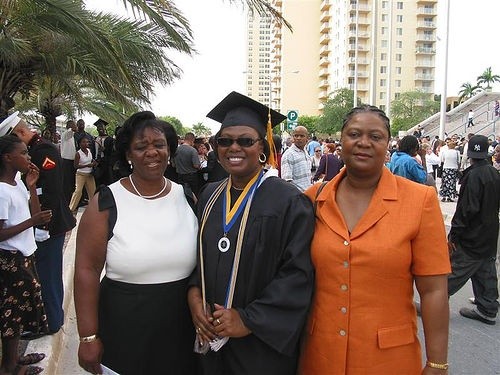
[[129, 174, 167, 198]]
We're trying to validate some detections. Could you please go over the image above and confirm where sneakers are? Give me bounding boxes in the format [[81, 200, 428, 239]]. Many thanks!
[[460, 307, 496, 326]]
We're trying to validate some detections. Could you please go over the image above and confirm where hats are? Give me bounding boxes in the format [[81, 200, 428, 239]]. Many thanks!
[[0, 110, 22, 137], [94, 119, 109, 126], [206, 91, 288, 167], [467, 135, 489, 159]]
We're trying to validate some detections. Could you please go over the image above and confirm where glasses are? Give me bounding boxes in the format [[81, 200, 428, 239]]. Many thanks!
[[219, 138, 262, 148], [315, 150, 321, 153]]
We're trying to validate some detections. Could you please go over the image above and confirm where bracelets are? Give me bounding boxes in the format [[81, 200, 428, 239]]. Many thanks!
[[80, 334, 97, 343], [426, 360, 448, 370]]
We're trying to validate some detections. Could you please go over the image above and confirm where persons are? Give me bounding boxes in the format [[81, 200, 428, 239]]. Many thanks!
[[75, 111, 199, 375], [385, 125, 475, 202], [42, 118, 122, 212], [495, 101, 499, 116], [415, 135, 500, 325], [467, 110, 474, 127], [487, 135, 500, 172], [302, 105, 452, 375], [0, 110, 77, 375], [187, 91, 317, 375], [273, 126, 344, 194], [167, 132, 230, 195]]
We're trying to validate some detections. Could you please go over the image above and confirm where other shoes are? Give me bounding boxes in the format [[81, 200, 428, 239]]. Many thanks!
[[447, 198, 454, 202], [441, 198, 446, 202]]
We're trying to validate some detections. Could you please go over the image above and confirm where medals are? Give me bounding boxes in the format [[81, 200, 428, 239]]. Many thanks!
[[218, 237, 230, 252]]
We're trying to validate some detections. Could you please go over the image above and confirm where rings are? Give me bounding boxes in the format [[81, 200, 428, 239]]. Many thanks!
[[198, 328, 200, 331], [217, 319, 220, 324]]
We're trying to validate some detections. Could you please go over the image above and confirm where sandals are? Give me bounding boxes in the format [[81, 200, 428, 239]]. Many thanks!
[[18, 353, 45, 364], [11, 366, 44, 375]]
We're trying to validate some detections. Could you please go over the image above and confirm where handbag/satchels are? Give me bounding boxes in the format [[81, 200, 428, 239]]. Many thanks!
[[437, 167, 443, 177], [314, 174, 328, 184], [457, 151, 460, 172]]
